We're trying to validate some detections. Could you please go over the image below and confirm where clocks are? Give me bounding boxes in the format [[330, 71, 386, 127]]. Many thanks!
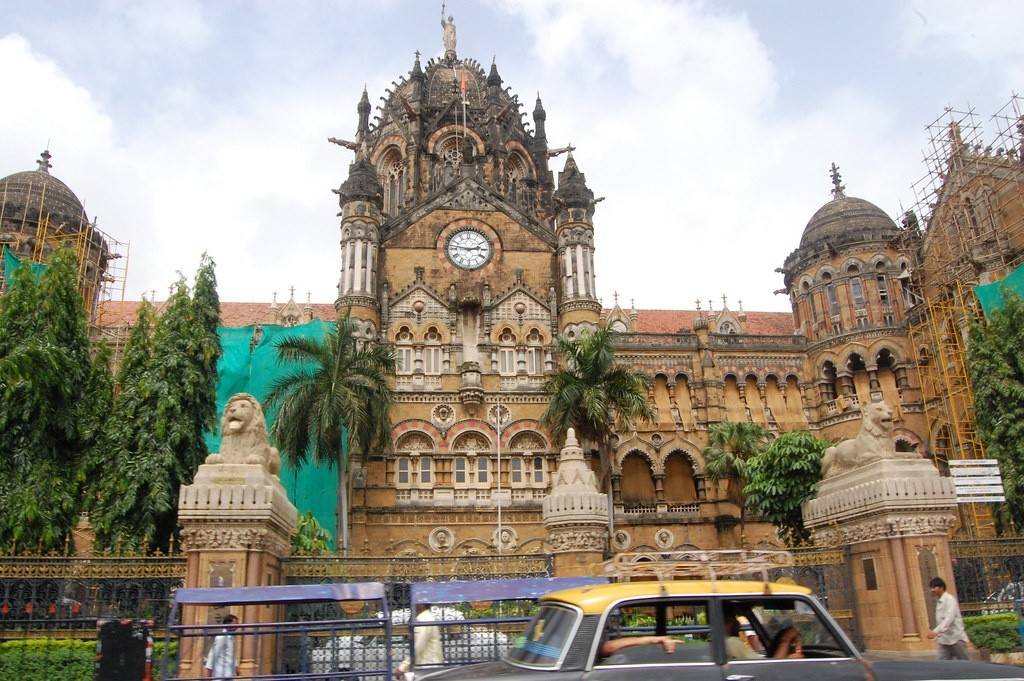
[[443, 225, 494, 272]]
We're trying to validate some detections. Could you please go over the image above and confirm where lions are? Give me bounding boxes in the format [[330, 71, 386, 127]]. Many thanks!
[[204, 392, 281, 476], [819, 400, 923, 480]]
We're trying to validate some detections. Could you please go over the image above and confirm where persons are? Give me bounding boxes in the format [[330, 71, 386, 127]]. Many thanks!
[[396, 604, 444, 681], [741, 608, 765, 651], [926, 577, 970, 660], [724, 613, 803, 659], [599, 607, 685, 657], [206, 614, 240, 681], [441, 14, 457, 50]]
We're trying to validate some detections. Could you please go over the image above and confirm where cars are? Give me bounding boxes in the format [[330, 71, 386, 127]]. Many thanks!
[[404, 579, 1024, 680]]
